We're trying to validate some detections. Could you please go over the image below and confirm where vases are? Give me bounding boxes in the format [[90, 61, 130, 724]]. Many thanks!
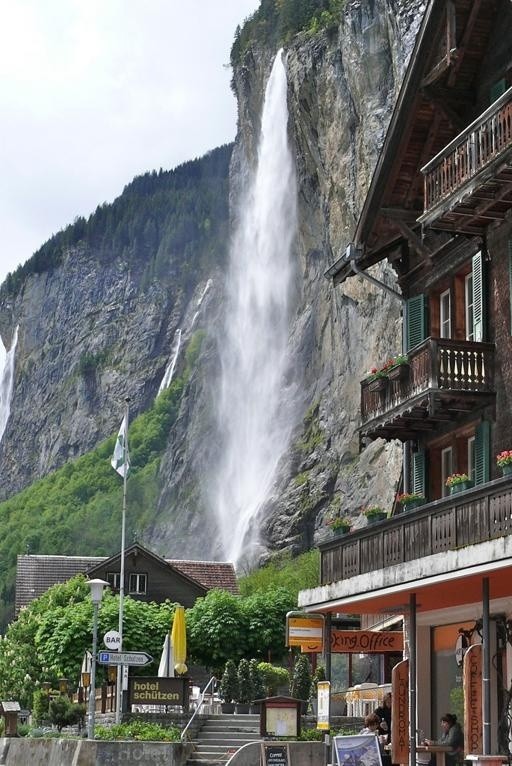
[[366, 512, 388, 524], [404, 498, 424, 510], [501, 464, 511, 476], [332, 525, 353, 535], [449, 481, 474, 494]]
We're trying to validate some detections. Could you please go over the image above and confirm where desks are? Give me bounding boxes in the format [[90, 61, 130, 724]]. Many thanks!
[[384, 744, 453, 766]]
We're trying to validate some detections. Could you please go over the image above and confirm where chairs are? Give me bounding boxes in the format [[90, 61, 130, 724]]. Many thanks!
[[346, 683, 392, 717]]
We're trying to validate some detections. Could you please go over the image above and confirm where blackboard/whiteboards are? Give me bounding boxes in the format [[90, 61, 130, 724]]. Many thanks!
[[260, 743, 291, 766]]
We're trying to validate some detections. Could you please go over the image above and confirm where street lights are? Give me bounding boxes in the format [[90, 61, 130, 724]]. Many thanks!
[[85, 578, 111, 739]]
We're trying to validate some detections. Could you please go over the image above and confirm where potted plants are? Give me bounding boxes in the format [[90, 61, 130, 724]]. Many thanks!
[[217, 657, 267, 714]]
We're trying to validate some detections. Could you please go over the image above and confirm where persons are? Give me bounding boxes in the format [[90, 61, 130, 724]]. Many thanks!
[[375, 692, 391, 745], [359, 714, 384, 755], [424, 712, 464, 766]]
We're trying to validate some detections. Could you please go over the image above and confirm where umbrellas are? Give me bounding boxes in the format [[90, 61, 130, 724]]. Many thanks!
[[81, 650, 93, 706], [158, 630, 175, 678], [111, 406, 130, 479], [171, 605, 188, 678]]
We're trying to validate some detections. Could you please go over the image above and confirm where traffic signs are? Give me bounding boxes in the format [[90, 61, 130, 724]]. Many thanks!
[[99, 650, 154, 667]]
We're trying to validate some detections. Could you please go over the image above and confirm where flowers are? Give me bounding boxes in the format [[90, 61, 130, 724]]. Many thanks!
[[326, 518, 350, 530], [445, 473, 469, 487], [496, 450, 512, 468], [366, 352, 409, 380], [361, 504, 382, 517], [395, 492, 422, 506]]
